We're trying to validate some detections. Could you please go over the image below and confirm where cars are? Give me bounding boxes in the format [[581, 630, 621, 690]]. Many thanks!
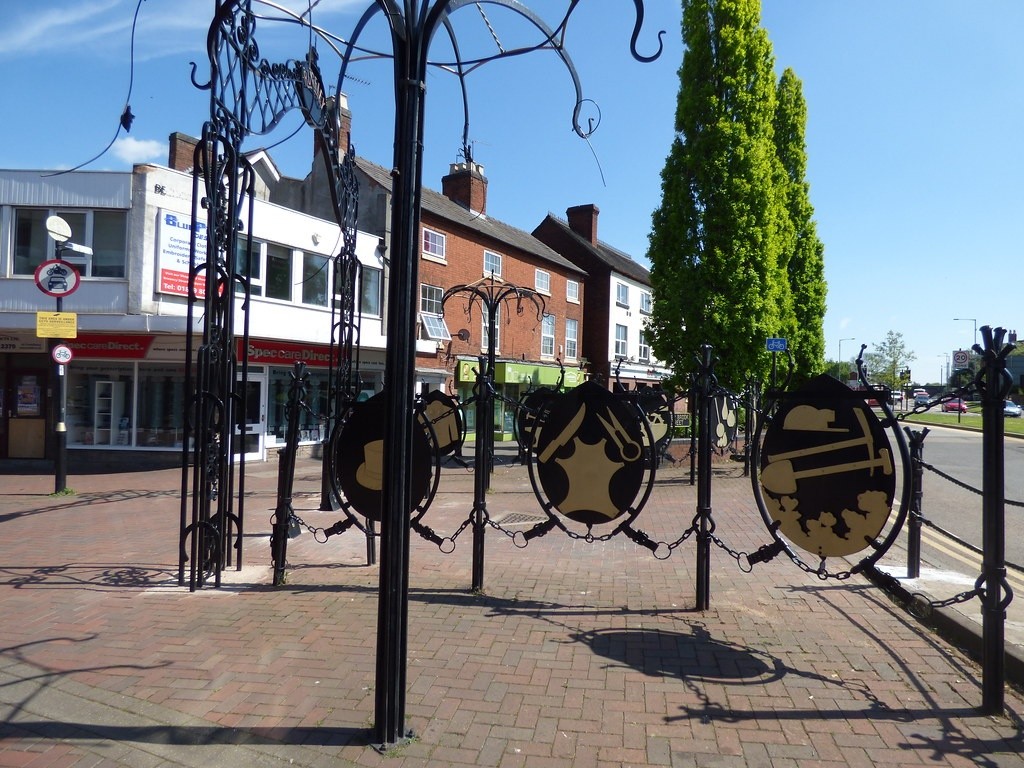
[[891, 388, 930, 407], [1004, 400, 1022, 416], [941, 397, 967, 413]]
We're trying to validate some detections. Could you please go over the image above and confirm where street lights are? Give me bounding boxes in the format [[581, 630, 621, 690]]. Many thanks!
[[953, 318, 977, 378], [838, 337, 855, 381]]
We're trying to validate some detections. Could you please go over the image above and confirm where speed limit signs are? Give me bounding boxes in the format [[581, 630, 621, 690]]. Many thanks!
[[952, 350, 969, 368]]
